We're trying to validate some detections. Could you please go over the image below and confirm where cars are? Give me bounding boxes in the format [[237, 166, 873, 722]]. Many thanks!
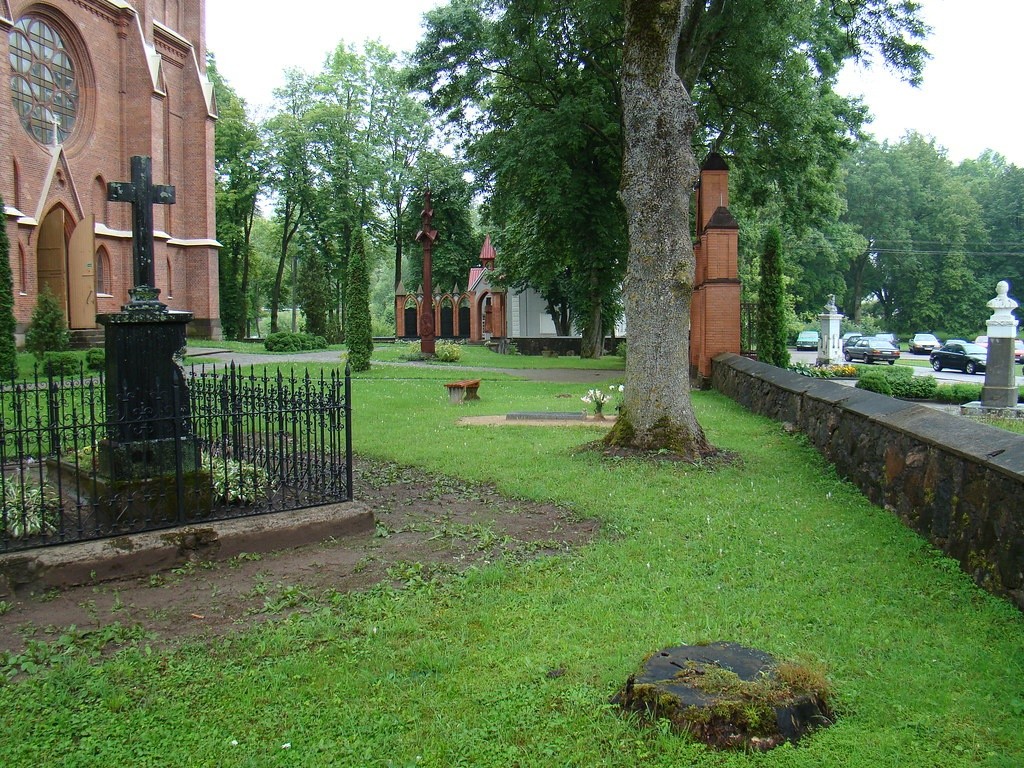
[[929, 341, 987, 374], [796, 331, 820, 351], [940, 335, 988, 349], [843, 336, 901, 365], [841, 333, 900, 347], [908, 333, 941, 354], [1015, 339, 1024, 364]]
